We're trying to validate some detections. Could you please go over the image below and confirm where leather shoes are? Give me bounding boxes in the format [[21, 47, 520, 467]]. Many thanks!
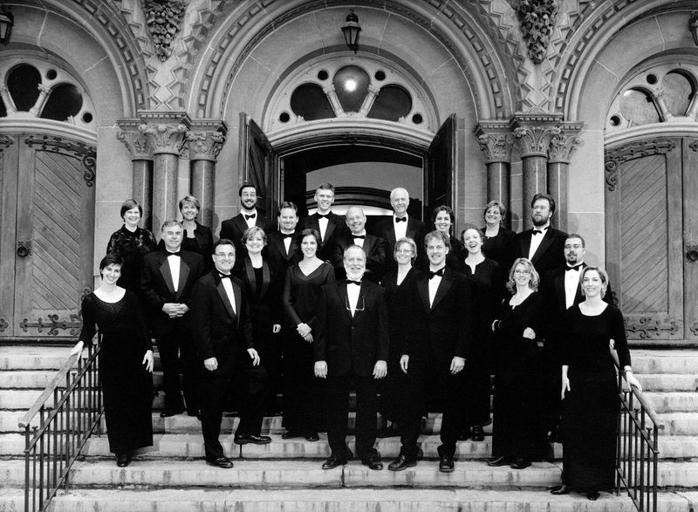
[[389, 453, 419, 473], [439, 454, 456, 474], [366, 456, 382, 472], [510, 457, 532, 468], [160, 405, 183, 417], [118, 450, 131, 467], [234, 433, 273, 446], [283, 429, 301, 439], [307, 429, 320, 443], [586, 486, 601, 503], [487, 454, 507, 466], [210, 452, 233, 470], [378, 426, 396, 438], [551, 483, 571, 496], [470, 423, 483, 443], [322, 447, 353, 469]]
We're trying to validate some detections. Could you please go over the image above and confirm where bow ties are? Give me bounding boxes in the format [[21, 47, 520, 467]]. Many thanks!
[[532, 225, 548, 237], [280, 231, 296, 238], [351, 234, 367, 241], [426, 268, 447, 278], [345, 279, 363, 286], [565, 265, 582, 272], [317, 214, 331, 221], [166, 251, 184, 259], [219, 271, 234, 280], [395, 214, 408, 225], [245, 212, 257, 223]]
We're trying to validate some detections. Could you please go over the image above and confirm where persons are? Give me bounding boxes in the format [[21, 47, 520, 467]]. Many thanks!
[[296, 182, 352, 261], [516, 192, 569, 294], [330, 206, 390, 285], [455, 226, 506, 442], [388, 230, 472, 472], [312, 245, 389, 471], [431, 204, 470, 271], [548, 233, 589, 442], [376, 236, 426, 438], [188, 238, 272, 469], [550, 265, 643, 501], [157, 195, 215, 276], [231, 226, 284, 417], [280, 228, 336, 442], [69, 253, 155, 467], [261, 200, 304, 278], [374, 187, 431, 268], [105, 198, 157, 339], [480, 199, 517, 274], [487, 257, 548, 468], [138, 219, 206, 417], [220, 182, 276, 259]]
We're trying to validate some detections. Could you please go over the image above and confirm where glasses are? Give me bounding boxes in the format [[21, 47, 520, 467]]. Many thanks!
[[514, 268, 530, 277], [218, 251, 236, 258]]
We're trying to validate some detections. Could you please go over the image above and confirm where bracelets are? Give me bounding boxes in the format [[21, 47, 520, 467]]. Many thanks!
[[624, 370, 634, 374]]
